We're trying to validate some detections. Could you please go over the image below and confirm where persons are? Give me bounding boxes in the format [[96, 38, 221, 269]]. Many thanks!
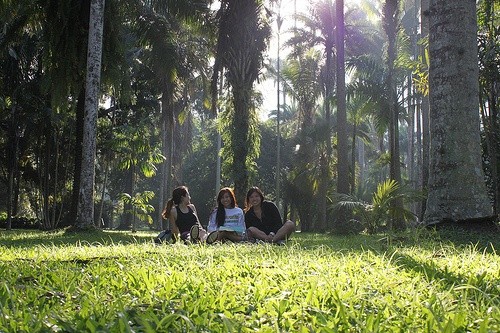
[[162, 186, 218, 244], [244, 187, 295, 246], [207, 187, 246, 245]]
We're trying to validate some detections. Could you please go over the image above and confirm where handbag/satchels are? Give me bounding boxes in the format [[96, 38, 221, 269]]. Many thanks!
[[155, 230, 176, 244]]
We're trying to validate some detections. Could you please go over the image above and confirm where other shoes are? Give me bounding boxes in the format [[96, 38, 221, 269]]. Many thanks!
[[277, 239, 285, 246], [190, 225, 199, 244], [206, 231, 218, 244]]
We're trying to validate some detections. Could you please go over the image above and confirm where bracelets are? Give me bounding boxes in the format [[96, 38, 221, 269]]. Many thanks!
[[269, 232, 275, 236]]
